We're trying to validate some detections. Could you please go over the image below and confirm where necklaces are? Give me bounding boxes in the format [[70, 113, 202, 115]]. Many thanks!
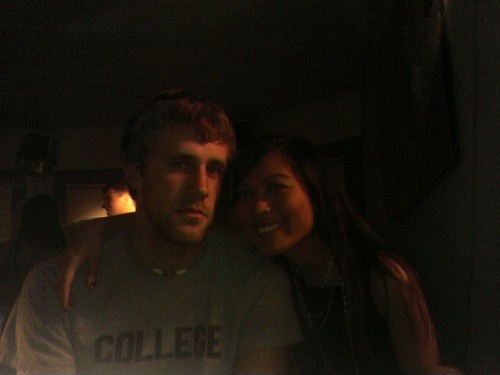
[[288, 254, 360, 375], [128, 220, 207, 276]]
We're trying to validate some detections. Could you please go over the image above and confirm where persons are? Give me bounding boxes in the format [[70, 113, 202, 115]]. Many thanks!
[[0, 194, 58, 338], [100, 175, 136, 217], [0, 84, 306, 375], [59, 133, 465, 375]]
